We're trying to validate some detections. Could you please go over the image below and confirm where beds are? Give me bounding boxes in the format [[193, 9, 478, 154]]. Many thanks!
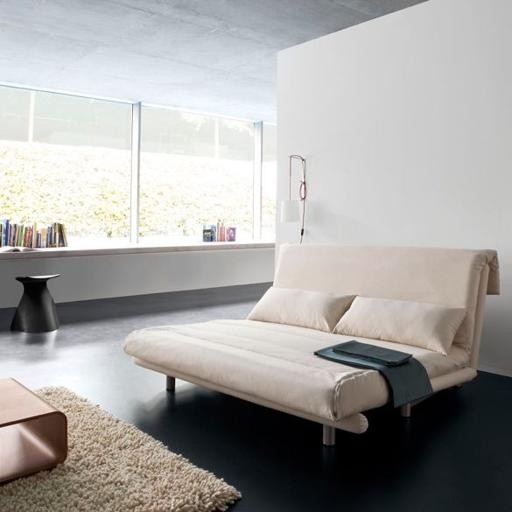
[[123, 244, 500, 445]]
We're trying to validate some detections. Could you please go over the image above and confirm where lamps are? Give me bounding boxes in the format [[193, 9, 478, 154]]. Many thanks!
[[281, 154, 306, 243]]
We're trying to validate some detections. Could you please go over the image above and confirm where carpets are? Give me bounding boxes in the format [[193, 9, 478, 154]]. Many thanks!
[[0, 385, 242, 512]]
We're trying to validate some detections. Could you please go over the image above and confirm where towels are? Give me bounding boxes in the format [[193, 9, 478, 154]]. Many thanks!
[[313, 341, 433, 408], [333, 340, 412, 366]]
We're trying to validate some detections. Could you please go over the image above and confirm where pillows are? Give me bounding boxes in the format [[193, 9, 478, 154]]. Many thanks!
[[332, 294, 466, 357], [247, 285, 357, 332]]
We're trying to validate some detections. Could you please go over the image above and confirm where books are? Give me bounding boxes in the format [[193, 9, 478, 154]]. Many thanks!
[[0, 217, 70, 252]]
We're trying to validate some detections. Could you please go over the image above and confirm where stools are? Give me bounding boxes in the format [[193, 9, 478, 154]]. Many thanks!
[[10, 272, 62, 332]]
